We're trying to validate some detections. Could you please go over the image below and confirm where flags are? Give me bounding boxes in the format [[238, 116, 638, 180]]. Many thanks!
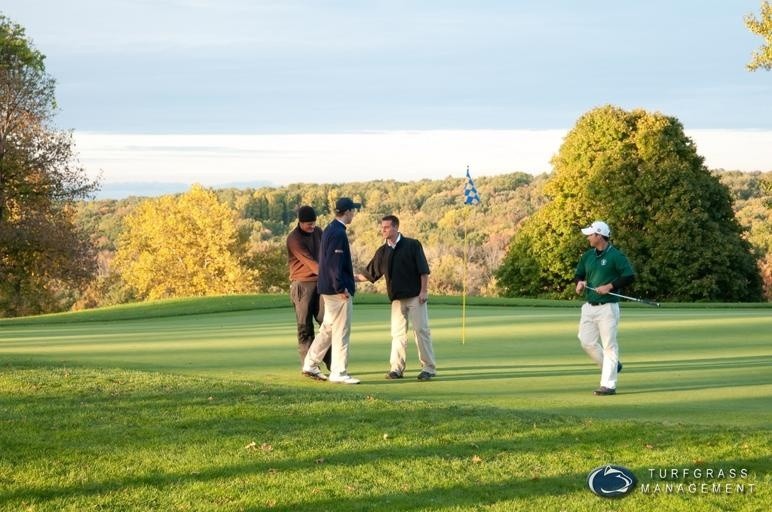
[[464, 170, 482, 206]]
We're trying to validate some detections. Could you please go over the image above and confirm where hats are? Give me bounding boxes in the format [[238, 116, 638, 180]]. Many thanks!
[[336, 198, 361, 212], [581, 220, 611, 238], [299, 206, 316, 222]]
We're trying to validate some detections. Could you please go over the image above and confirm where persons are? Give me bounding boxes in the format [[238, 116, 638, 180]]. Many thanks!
[[286, 205, 331, 373], [301, 198, 359, 384], [352, 215, 438, 379], [576, 219, 637, 395]]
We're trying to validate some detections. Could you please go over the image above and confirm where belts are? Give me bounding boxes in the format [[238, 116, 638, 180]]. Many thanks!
[[589, 301, 605, 305]]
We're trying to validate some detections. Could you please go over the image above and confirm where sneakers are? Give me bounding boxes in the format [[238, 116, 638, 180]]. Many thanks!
[[329, 376, 360, 383], [417, 371, 435, 379], [304, 366, 327, 380], [385, 372, 402, 379]]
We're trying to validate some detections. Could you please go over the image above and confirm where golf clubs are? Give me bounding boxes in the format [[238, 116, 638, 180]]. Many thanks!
[[584, 285, 660, 308]]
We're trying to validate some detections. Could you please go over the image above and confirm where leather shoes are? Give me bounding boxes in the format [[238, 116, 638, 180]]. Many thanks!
[[618, 361, 622, 372], [594, 387, 615, 395]]
